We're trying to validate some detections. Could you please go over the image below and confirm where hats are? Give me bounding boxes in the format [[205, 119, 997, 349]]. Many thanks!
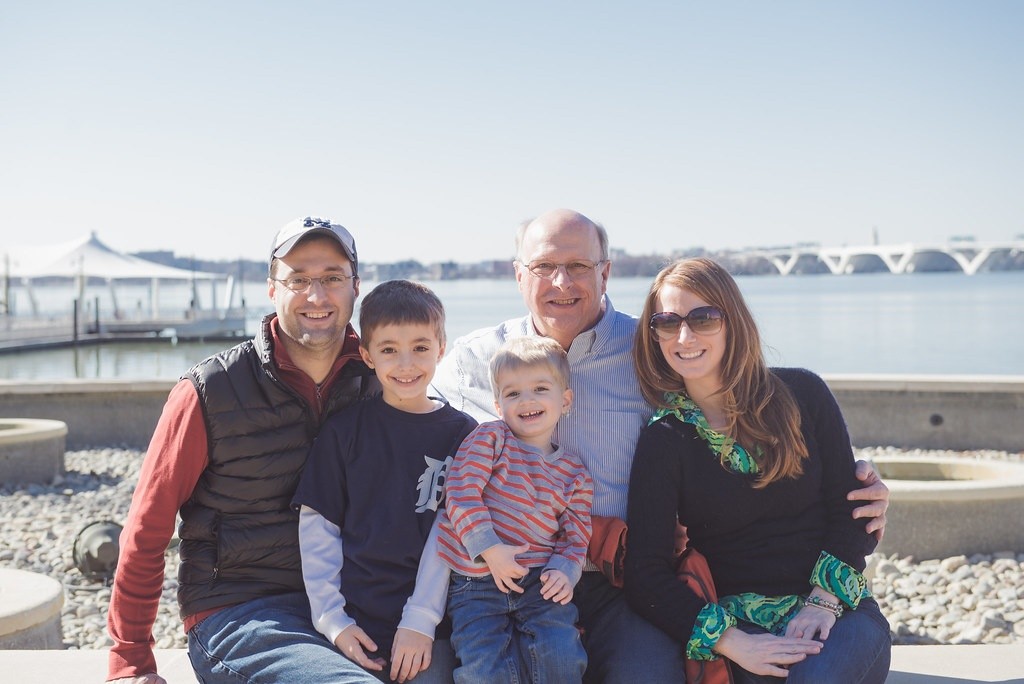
[[268, 216, 358, 277]]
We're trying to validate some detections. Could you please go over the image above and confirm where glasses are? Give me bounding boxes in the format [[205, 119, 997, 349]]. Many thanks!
[[270, 275, 356, 290], [650, 306, 726, 342], [518, 257, 605, 277]]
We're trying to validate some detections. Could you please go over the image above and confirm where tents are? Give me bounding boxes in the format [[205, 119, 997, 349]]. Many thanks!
[[0, 231, 238, 325]]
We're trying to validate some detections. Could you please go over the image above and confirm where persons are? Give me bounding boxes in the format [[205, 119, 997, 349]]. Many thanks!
[[624, 252, 893, 683], [106, 213, 394, 684], [290, 276, 479, 684], [431, 335, 595, 684], [429, 206, 890, 684]]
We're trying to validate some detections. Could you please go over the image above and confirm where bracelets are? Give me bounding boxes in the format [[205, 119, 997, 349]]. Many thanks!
[[800, 594, 845, 621]]
